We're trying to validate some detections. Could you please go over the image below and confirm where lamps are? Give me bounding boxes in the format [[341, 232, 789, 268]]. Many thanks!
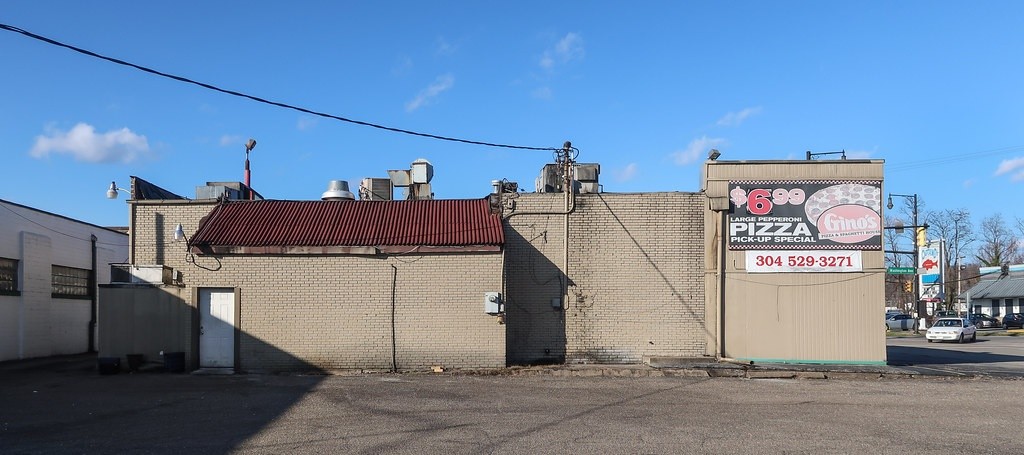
[[708, 149, 721, 161], [174, 223, 188, 245], [107, 181, 130, 199]]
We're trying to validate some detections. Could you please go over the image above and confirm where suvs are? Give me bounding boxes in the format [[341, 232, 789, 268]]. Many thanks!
[[1002, 313, 1024, 330]]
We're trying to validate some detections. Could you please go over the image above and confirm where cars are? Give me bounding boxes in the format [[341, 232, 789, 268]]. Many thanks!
[[926, 318, 977, 344], [886, 313, 920, 330], [886, 312, 901, 319], [965, 313, 999, 329], [935, 310, 957, 326]]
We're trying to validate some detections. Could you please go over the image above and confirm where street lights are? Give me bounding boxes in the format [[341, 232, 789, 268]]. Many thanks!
[[886, 192, 920, 334]]
